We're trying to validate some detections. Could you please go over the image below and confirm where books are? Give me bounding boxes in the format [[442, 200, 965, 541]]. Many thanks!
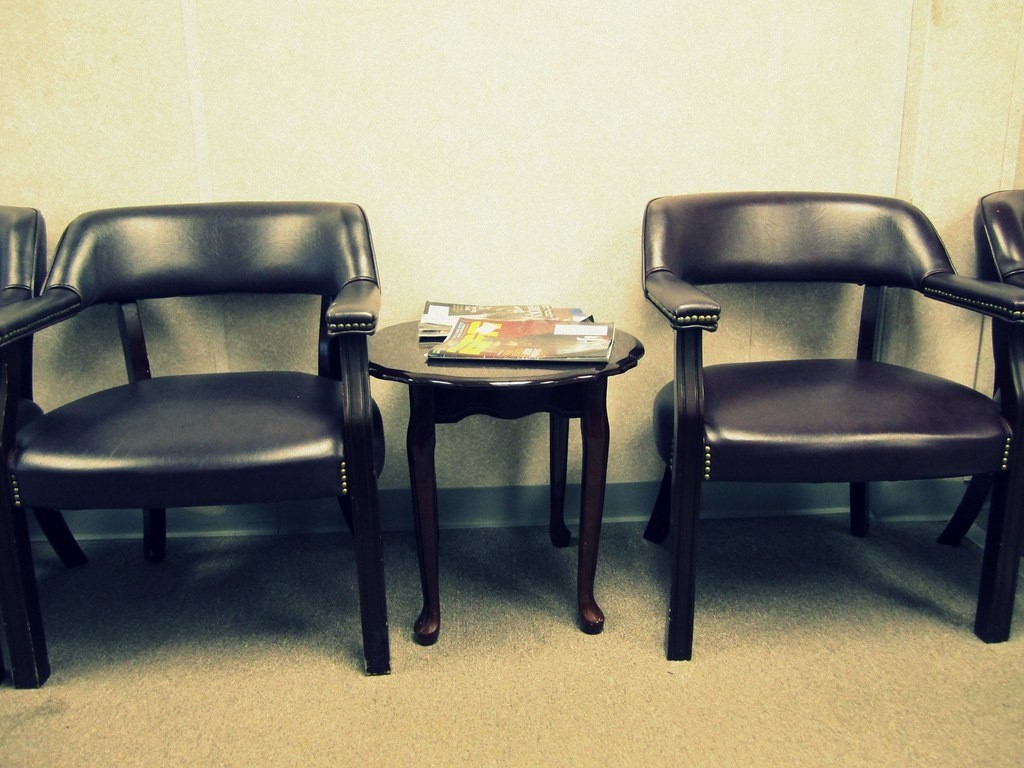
[[426, 316, 615, 363], [418, 300, 594, 342]]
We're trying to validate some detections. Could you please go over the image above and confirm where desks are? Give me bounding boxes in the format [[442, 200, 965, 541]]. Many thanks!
[[365, 315, 645, 646]]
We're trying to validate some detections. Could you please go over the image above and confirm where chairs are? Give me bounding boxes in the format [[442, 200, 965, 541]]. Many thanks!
[[938, 188, 1024, 545], [640, 193, 1024, 662], [0, 201, 392, 688], [0, 206, 98, 572]]
